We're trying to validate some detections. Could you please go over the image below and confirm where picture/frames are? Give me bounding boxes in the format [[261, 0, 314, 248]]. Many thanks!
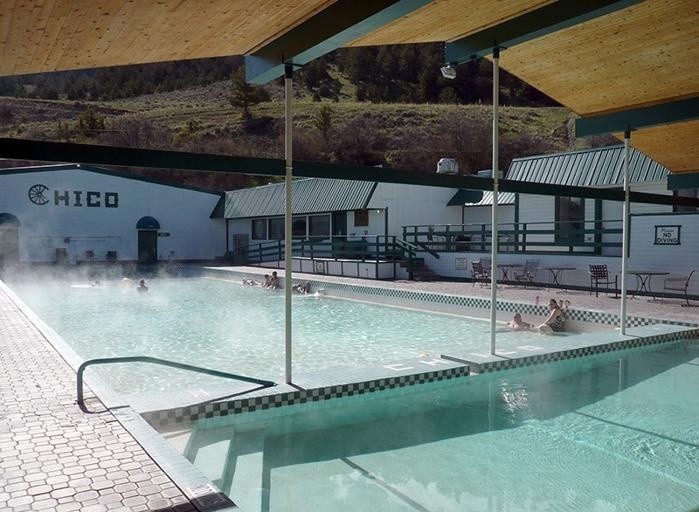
[[653, 225, 682, 245]]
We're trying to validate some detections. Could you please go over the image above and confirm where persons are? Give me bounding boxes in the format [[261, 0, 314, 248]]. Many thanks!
[[262, 274, 271, 289], [136, 279, 148, 294], [505, 313, 535, 331], [267, 271, 279, 291], [292, 280, 312, 296], [538, 298, 565, 334], [242, 278, 260, 287]]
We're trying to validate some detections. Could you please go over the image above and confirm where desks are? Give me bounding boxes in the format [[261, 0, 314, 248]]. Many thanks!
[[79, 255, 102, 264]]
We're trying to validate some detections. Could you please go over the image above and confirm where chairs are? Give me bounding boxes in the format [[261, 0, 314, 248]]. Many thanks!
[[54, 248, 69, 265], [470, 257, 695, 307], [105, 251, 119, 262]]
